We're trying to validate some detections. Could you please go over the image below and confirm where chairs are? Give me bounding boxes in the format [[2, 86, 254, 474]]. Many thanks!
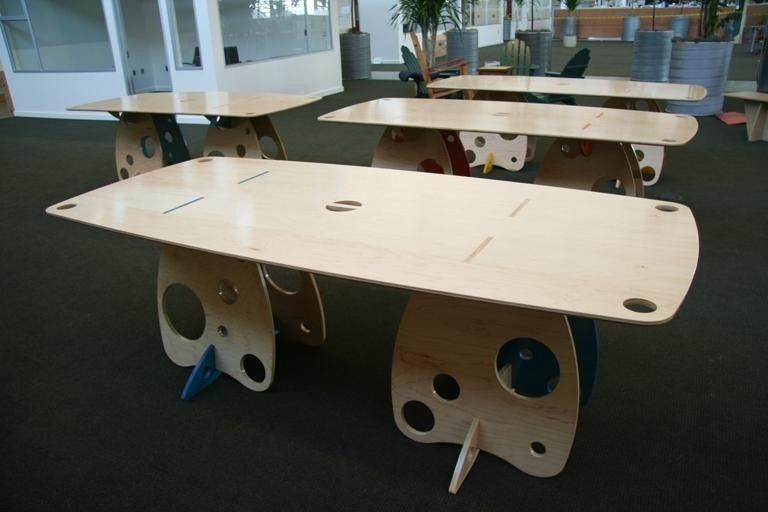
[[410, 30, 476, 100]]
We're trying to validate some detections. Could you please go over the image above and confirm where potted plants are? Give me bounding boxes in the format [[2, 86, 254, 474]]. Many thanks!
[[559, 0, 586, 48], [665, 1, 744, 116]]
